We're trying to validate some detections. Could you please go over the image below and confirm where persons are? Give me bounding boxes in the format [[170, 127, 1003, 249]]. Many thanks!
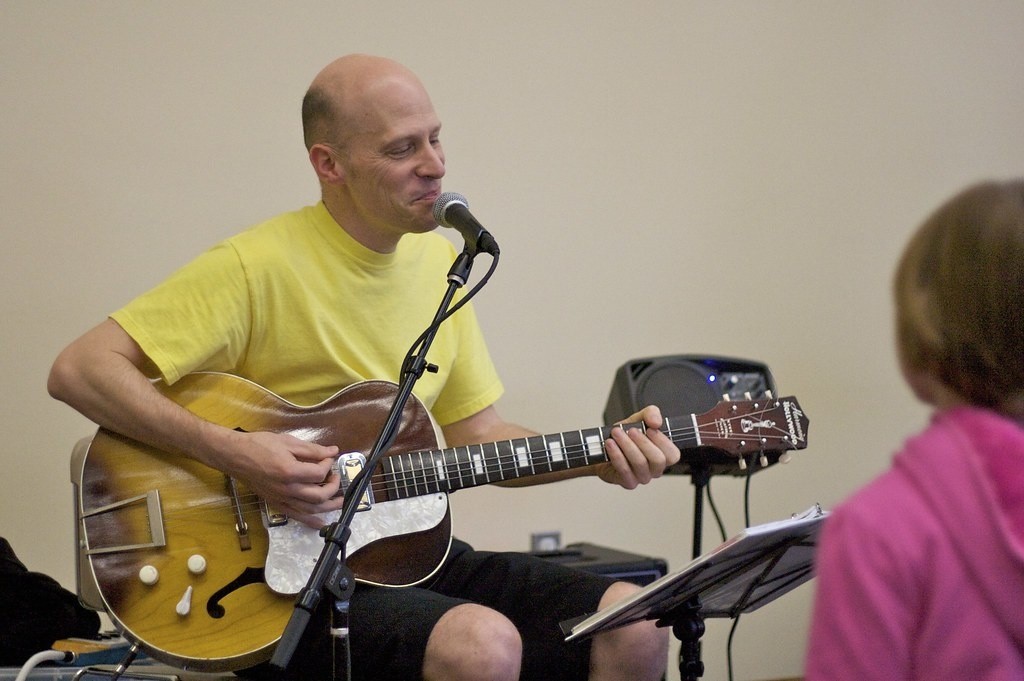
[[805, 181, 1024, 681], [45, 55, 669, 681]]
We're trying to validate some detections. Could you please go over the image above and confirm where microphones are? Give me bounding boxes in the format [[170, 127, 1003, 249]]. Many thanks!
[[432, 192, 500, 255]]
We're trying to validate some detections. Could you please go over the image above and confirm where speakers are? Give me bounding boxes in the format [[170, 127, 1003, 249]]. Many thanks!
[[604, 354, 782, 477]]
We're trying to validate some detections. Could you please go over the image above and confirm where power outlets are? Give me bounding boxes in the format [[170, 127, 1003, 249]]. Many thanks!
[[531, 531, 562, 555]]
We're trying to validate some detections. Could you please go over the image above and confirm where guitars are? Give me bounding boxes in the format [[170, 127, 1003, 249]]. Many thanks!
[[71, 371, 810, 671]]
